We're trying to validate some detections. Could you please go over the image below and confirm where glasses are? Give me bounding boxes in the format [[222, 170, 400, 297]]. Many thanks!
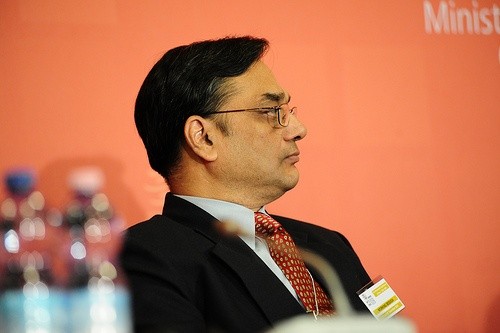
[[208, 103, 297, 127]]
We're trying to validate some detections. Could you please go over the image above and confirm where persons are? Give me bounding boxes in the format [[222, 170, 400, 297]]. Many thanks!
[[119, 34, 399, 333]]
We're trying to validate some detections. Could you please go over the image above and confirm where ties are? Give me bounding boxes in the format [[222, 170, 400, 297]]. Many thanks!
[[254, 212, 336, 318]]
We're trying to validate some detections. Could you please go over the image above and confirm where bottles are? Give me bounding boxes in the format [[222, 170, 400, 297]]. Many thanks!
[[60, 167, 133, 333], [0, 167, 60, 333]]
